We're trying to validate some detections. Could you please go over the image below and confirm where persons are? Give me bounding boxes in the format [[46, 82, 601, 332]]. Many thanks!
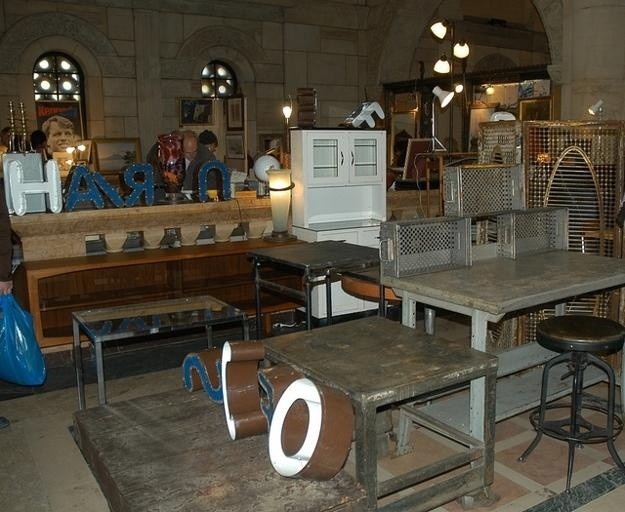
[[0, 177, 14, 428], [183, 130, 220, 190], [0, 126, 12, 178], [41, 115, 73, 159], [29, 130, 52, 161]]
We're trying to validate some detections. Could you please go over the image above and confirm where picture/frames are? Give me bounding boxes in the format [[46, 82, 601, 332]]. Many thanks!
[[33, 98, 84, 161], [224, 133, 245, 161], [89, 134, 144, 178], [224, 91, 246, 133], [177, 95, 216, 128], [402, 135, 435, 181]]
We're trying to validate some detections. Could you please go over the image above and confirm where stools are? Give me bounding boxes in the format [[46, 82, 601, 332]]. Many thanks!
[[518, 311, 624, 495]]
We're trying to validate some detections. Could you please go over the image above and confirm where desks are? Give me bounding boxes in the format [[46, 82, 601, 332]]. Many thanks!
[[243, 241, 386, 344], [254, 315, 499, 512], [70, 294, 248, 410], [377, 250, 624, 473]]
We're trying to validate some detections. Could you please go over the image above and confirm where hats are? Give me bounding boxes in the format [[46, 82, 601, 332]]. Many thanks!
[[199, 130, 218, 145]]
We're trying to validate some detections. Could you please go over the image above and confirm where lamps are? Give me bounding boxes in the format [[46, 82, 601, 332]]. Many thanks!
[[586, 97, 609, 153], [266, 165, 297, 243], [423, 12, 496, 157]]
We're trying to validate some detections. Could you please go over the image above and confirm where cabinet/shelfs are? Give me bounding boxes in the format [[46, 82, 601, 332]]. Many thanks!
[[292, 217, 398, 326], [288, 124, 389, 230], [18, 232, 311, 351]]
